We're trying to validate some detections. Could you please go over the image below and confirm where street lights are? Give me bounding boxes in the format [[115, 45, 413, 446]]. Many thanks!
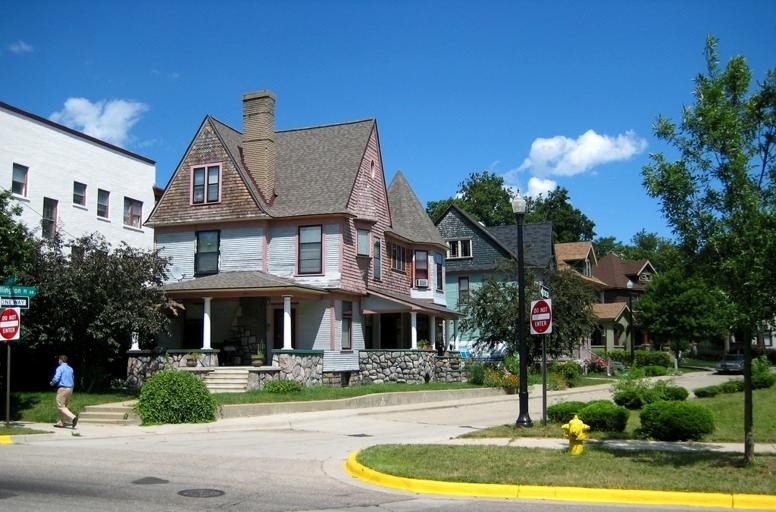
[[511, 189, 531, 424]]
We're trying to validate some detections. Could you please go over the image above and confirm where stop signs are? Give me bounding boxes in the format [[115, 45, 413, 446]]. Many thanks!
[[529, 300, 553, 335], [1, 310, 21, 340]]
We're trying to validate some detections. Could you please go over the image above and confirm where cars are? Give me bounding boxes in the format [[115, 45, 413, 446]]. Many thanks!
[[715, 354, 745, 374]]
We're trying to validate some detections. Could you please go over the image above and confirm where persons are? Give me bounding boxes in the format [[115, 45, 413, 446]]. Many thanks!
[[688, 340, 697, 357], [50, 355, 78, 428]]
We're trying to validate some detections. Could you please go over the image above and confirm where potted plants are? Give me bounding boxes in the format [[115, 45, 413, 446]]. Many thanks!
[[251, 339, 266, 366], [186, 352, 204, 366], [418, 339, 433, 349]]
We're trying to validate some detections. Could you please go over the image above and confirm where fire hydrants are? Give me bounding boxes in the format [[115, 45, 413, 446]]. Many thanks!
[[560, 413, 590, 452]]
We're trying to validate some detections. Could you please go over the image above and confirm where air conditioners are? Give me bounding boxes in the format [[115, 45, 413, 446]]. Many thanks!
[[418, 279, 428, 287]]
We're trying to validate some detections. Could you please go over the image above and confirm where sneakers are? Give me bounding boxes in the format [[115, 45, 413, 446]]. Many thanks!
[[54, 417, 78, 429]]
